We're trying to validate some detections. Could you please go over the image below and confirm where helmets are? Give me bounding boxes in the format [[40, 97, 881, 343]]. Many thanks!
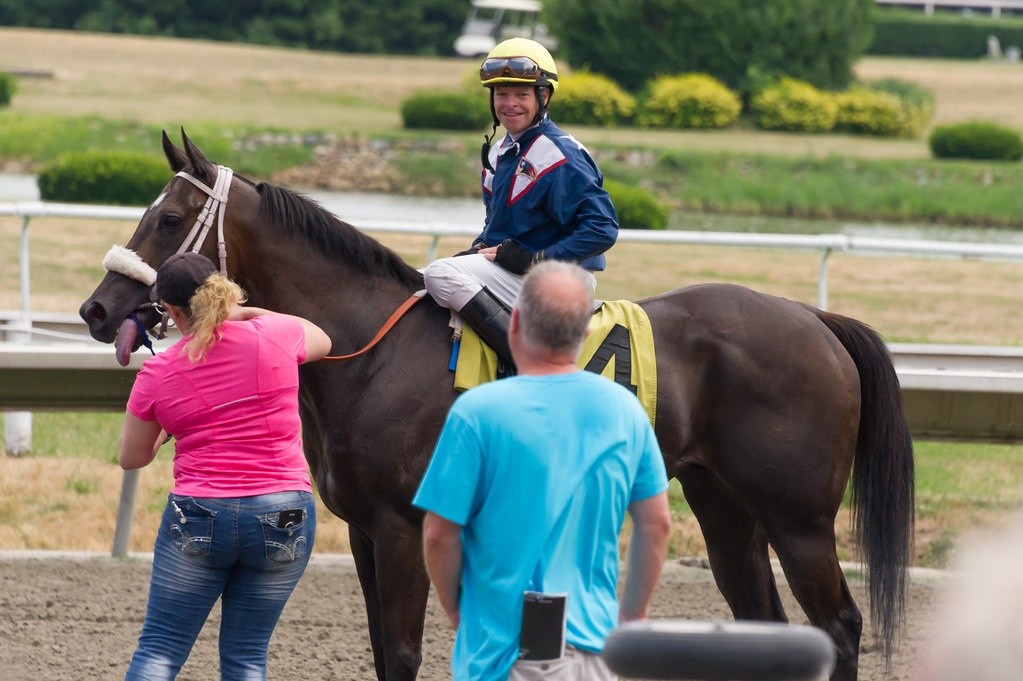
[[480, 38, 558, 93]]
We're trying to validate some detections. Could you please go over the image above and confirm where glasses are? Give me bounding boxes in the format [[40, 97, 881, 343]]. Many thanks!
[[480, 55, 557, 78]]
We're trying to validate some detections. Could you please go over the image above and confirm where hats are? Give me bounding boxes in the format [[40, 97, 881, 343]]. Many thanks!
[[149, 251, 217, 307]]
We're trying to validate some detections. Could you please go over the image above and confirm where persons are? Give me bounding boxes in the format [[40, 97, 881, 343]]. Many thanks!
[[424, 36, 620, 368], [120, 252, 333, 681], [412, 259, 672, 681]]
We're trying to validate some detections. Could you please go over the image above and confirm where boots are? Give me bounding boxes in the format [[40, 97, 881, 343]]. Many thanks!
[[458, 287, 516, 368]]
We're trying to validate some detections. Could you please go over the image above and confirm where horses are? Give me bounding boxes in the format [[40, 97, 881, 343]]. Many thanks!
[[78, 125, 917, 681]]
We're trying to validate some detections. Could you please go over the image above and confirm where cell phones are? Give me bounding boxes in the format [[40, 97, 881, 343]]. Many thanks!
[[277, 508, 303, 529]]
